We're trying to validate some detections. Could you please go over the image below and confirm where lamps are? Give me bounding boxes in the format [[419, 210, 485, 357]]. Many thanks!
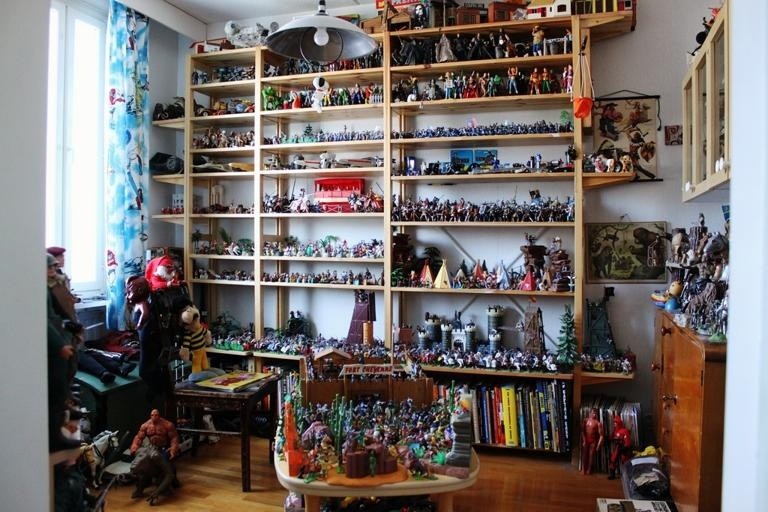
[[265, 0, 380, 67]]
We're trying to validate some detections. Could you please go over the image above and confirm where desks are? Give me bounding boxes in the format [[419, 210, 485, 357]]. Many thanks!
[[274, 411, 481, 512]]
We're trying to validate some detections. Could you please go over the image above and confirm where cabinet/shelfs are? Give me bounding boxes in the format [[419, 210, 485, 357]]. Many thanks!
[[680, 0, 730, 203], [151, 0, 636, 466], [650, 307, 727, 512]]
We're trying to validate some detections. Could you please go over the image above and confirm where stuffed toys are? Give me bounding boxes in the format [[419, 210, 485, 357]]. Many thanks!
[[47, 246, 213, 512]]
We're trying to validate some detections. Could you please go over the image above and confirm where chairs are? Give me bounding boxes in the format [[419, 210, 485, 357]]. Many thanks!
[[151, 283, 283, 492]]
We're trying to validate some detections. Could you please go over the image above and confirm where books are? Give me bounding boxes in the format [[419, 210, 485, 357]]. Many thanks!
[[433, 380, 569, 452], [578, 398, 641, 477]]
